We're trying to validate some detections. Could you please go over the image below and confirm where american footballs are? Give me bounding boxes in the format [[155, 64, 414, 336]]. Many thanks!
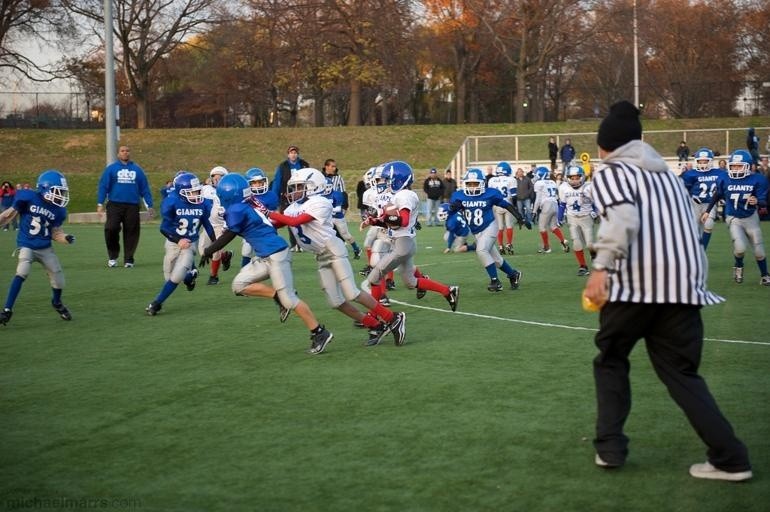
[[384, 202, 401, 231]]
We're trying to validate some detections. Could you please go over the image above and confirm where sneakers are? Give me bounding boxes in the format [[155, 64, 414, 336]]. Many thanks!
[[500, 243, 514, 255], [187, 250, 232, 290], [444, 286, 459, 311], [0, 309, 12, 326], [690, 461, 752, 481], [538, 240, 569, 253], [108, 260, 133, 268], [52, 299, 71, 319], [735, 267, 770, 285], [354, 266, 406, 347], [577, 266, 589, 275], [306, 330, 333, 354], [280, 306, 290, 322], [146, 303, 160, 315], [488, 271, 521, 291], [417, 275, 429, 299]]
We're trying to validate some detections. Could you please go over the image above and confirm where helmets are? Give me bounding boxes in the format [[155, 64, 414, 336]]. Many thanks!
[[363, 161, 414, 192], [695, 148, 714, 172], [37, 170, 69, 208], [173, 166, 269, 205], [287, 167, 334, 204], [728, 150, 753, 179], [462, 162, 585, 196]]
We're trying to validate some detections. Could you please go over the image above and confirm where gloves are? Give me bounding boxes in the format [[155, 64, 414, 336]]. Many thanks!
[[199, 249, 212, 268], [450, 199, 465, 212], [518, 218, 532, 230], [248, 193, 269, 218]]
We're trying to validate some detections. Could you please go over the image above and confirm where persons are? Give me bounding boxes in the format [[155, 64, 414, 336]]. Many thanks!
[[1, 181, 33, 231], [98, 145, 153, 268], [585, 100, 754, 480], [676, 127, 770, 286], [1, 170, 76, 324], [146, 145, 460, 356], [423, 163, 524, 292], [486, 136, 602, 276]]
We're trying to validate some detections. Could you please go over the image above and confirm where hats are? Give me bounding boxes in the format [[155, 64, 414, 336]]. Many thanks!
[[288, 146, 298, 152], [430, 169, 436, 173], [597, 101, 642, 152]]
